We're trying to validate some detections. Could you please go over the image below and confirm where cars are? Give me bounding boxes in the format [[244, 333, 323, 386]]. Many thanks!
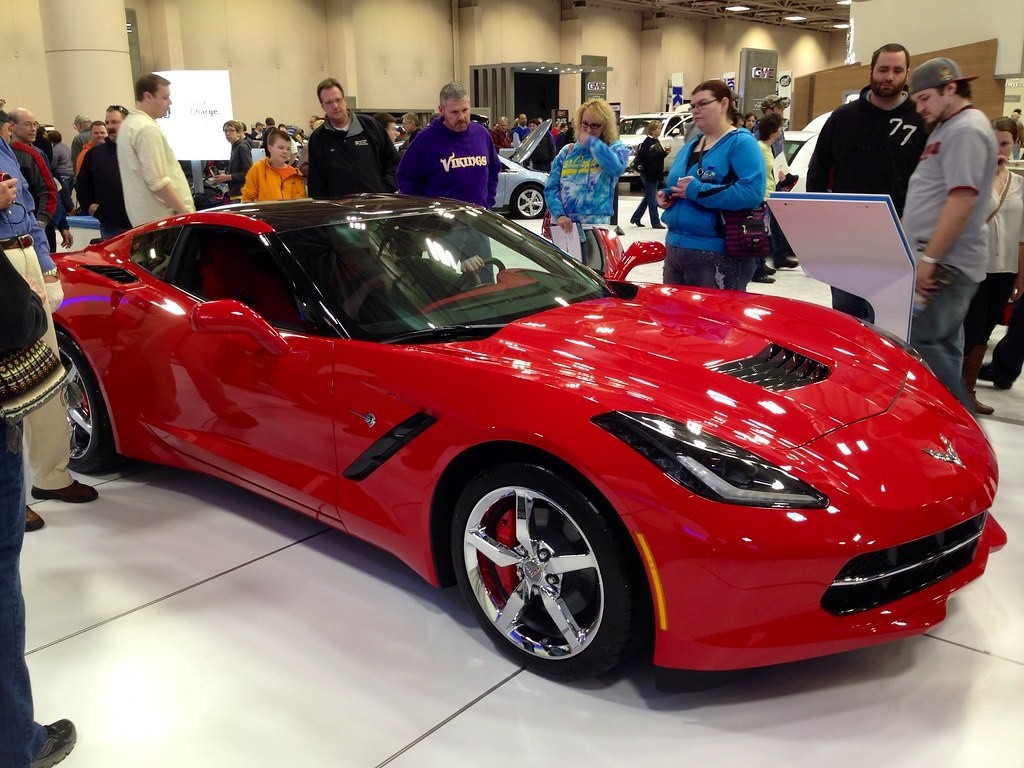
[[782, 110, 834, 193], [390, 116, 552, 221], [619, 110, 697, 179]]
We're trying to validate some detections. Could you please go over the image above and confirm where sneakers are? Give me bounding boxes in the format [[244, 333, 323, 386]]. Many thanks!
[[977, 361, 1014, 390]]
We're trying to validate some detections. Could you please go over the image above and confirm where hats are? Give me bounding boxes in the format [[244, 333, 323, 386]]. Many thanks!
[[761, 95, 791, 109], [255, 122, 265, 126], [908, 57, 979, 96]]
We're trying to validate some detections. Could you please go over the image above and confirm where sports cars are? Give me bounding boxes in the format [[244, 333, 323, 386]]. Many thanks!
[[39, 186, 1008, 685]]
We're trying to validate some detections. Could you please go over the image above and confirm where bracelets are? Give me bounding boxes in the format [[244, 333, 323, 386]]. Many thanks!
[[921, 254, 942, 268]]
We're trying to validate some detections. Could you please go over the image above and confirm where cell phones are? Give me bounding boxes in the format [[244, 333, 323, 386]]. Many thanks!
[[210, 166, 220, 177]]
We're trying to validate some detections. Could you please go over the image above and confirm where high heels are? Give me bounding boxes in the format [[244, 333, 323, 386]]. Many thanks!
[[652, 223, 666, 229], [631, 217, 645, 227]]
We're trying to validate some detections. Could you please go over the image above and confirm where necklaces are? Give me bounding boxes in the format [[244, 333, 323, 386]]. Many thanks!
[[994, 168, 1008, 186], [704, 125, 732, 146]]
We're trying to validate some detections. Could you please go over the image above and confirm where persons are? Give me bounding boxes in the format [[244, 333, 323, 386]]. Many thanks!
[[0, 266, 76, 768], [0, 113, 99, 534], [0, 100, 329, 255], [116, 74, 196, 230], [630, 121, 671, 230], [660, 80, 766, 290], [728, 94, 800, 284], [339, 224, 485, 329], [367, 110, 576, 175], [306, 78, 401, 201], [399, 83, 500, 286], [544, 98, 628, 258], [970, 108, 1024, 415], [900, 57, 997, 409], [805, 42, 933, 323]]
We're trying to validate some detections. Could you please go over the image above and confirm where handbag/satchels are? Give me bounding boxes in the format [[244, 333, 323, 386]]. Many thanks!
[[630, 155, 649, 174], [722, 209, 770, 256], [541, 209, 551, 239]]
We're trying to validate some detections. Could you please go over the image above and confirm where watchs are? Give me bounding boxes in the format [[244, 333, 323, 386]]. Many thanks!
[[42, 267, 61, 279]]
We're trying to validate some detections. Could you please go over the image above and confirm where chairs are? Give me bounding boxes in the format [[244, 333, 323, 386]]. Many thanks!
[[200, 233, 321, 335]]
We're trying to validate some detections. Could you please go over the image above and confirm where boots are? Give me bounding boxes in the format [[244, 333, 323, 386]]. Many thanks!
[[963, 345, 994, 416]]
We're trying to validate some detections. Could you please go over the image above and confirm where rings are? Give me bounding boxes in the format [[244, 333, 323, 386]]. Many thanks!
[[564, 224, 566, 227]]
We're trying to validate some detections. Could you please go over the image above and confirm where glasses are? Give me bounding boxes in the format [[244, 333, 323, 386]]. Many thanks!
[[14, 122, 40, 129], [580, 117, 602, 129], [112, 105, 128, 115], [323, 98, 344, 105], [688, 98, 721, 112], [224, 129, 237, 132], [74, 124, 78, 129]]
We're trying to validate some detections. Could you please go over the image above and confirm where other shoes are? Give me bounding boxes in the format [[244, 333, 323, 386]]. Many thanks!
[[774, 260, 798, 269], [751, 275, 775, 283], [763, 264, 776, 274]]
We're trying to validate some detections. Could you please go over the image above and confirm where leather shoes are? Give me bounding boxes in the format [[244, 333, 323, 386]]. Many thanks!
[[25, 505, 45, 531], [31, 480, 99, 502], [29, 719, 77, 768]]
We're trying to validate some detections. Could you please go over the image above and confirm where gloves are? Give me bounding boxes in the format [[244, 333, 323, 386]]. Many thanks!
[[44, 280, 65, 314]]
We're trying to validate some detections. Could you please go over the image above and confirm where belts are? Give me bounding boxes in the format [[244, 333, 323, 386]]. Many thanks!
[[0, 234, 35, 249]]
